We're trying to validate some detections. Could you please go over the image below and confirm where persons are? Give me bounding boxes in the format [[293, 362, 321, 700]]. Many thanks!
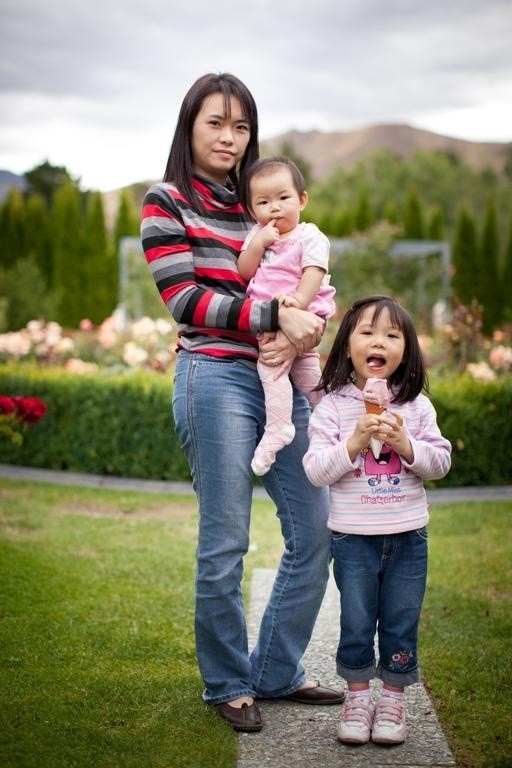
[[223, 154, 338, 480], [300, 292, 456, 749], [137, 70, 351, 738]]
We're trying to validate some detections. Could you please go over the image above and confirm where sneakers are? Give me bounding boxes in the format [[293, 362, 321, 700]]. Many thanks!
[[337, 695, 375, 744], [371, 694, 406, 744]]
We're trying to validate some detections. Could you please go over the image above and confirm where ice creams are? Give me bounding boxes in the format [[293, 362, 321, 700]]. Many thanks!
[[362, 377, 391, 416]]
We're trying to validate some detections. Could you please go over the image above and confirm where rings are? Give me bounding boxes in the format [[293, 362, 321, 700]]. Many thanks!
[[271, 358, 280, 366]]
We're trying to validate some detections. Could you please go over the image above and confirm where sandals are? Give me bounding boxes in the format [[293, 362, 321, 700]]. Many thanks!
[[215, 699, 263, 732], [280, 680, 346, 704]]
